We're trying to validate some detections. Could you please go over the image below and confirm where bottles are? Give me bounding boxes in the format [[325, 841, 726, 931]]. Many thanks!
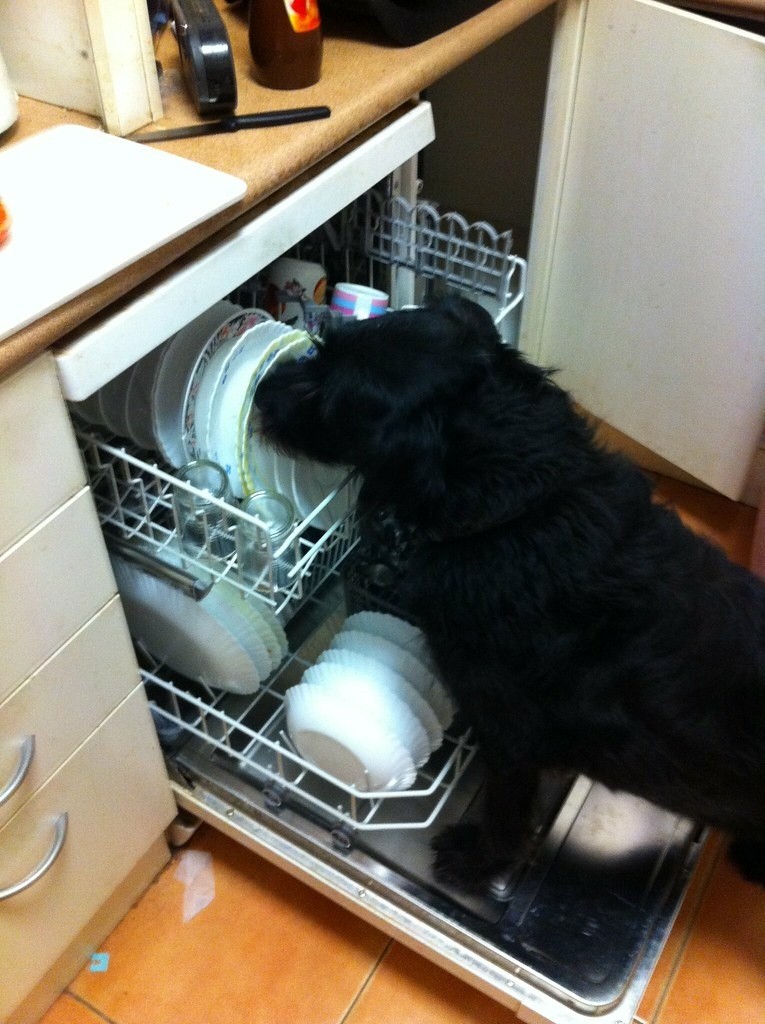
[[245, 1, 323, 92]]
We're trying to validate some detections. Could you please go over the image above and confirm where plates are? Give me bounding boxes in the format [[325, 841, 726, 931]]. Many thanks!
[[63, 290, 367, 538], [100, 546, 458, 797]]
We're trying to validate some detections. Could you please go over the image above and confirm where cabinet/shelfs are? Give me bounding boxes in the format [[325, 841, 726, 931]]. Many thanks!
[[0, 0, 765, 1024]]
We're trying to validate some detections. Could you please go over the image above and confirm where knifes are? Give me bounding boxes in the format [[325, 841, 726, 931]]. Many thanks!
[[125, 106, 332, 142]]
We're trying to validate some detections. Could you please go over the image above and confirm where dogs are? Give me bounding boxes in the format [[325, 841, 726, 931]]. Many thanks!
[[250, 275, 765, 889]]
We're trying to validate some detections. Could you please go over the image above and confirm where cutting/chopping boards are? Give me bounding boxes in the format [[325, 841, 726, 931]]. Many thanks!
[[0, 125, 249, 341]]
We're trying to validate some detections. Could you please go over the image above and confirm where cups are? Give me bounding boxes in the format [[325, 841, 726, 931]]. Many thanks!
[[235, 490, 302, 591], [332, 282, 392, 325], [171, 459, 238, 564], [263, 256, 326, 337]]
